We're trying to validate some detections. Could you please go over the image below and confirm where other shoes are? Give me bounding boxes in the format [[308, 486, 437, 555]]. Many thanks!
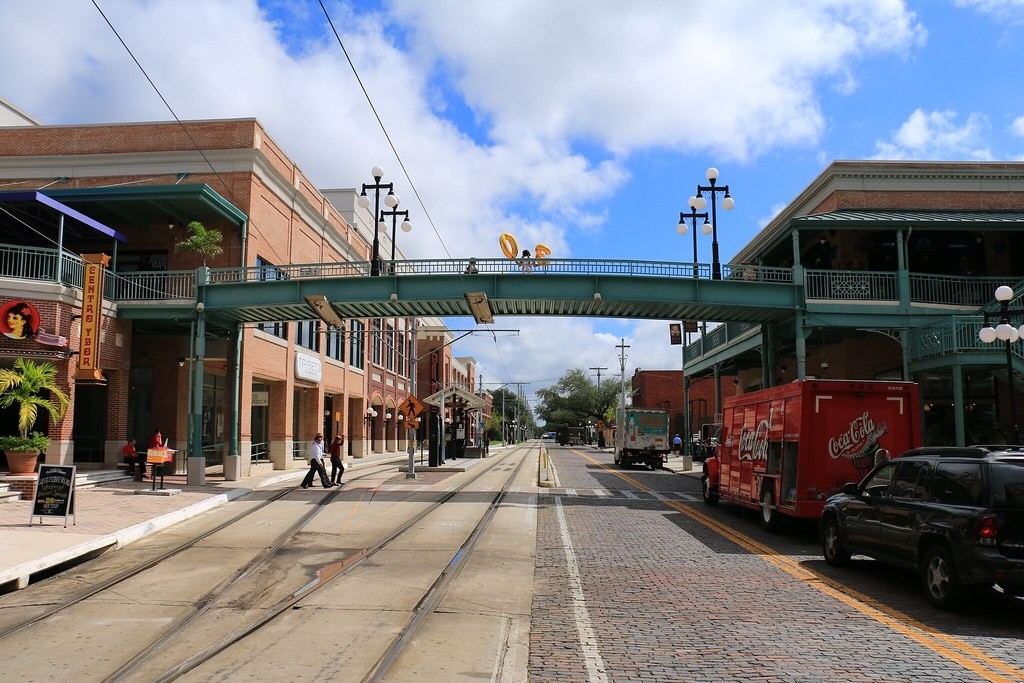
[[308, 484, 312, 487], [301, 484, 307, 488], [324, 485, 332, 488], [329, 482, 335, 485], [336, 481, 343, 484]]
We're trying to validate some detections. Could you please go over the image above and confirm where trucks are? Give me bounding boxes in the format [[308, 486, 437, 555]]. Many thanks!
[[700, 377, 922, 536], [613, 407, 672, 471]]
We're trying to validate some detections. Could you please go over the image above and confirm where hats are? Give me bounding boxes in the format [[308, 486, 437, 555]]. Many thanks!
[[316, 433, 324, 437]]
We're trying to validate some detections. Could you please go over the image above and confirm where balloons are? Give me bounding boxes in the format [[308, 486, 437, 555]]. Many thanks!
[[500, 233, 518, 258], [536, 245, 551, 265]]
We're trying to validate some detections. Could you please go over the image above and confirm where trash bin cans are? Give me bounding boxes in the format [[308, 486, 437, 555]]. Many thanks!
[[157, 450, 176, 475]]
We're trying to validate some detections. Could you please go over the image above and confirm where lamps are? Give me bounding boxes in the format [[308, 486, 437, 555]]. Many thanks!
[[396, 415, 403, 425], [364, 407, 378, 426], [384, 413, 392, 423], [445, 418, 450, 425], [324, 410, 331, 420], [820, 237, 826, 244], [781, 366, 786, 372], [179, 357, 185, 366], [733, 380, 738, 385], [821, 337, 828, 370], [168, 222, 174, 229]]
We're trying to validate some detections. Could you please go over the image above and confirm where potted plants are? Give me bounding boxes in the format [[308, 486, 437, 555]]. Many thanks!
[[0, 358, 71, 474], [172, 222, 222, 284]]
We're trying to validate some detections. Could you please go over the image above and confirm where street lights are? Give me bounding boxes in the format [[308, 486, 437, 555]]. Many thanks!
[[378, 192, 412, 276], [693, 167, 737, 280], [978, 285, 1023, 448], [360, 164, 402, 276], [669, 208, 712, 278]]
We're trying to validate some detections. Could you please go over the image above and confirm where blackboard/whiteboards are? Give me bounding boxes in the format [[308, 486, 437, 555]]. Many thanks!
[[32, 464, 77, 516]]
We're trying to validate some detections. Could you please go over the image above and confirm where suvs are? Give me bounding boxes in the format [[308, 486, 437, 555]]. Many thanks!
[[822, 445, 1024, 610]]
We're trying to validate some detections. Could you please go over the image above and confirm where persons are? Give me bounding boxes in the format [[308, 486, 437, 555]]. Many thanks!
[[579, 430, 585, 443], [123, 438, 148, 478], [700, 437, 715, 474], [464, 256, 479, 274], [598, 429, 606, 453], [673, 434, 682, 458], [150, 427, 163, 448], [329, 435, 345, 486], [508, 430, 511, 437], [300, 433, 338, 489], [514, 250, 540, 275]]
